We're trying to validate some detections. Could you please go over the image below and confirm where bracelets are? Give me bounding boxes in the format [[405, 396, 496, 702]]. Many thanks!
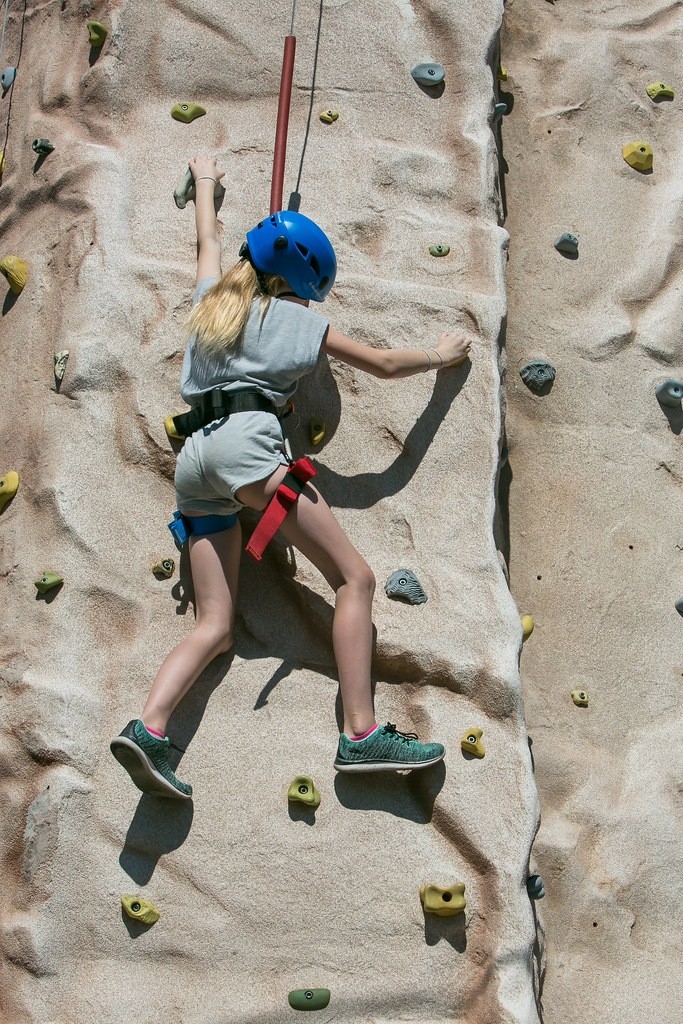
[[195, 176, 216, 185], [431, 348, 443, 369], [421, 350, 432, 372]]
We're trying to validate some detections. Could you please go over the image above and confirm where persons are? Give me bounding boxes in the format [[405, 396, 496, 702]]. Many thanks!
[[110, 156, 474, 799]]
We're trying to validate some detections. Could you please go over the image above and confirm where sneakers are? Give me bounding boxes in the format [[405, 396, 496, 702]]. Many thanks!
[[333, 722, 446, 774], [110, 719, 193, 799]]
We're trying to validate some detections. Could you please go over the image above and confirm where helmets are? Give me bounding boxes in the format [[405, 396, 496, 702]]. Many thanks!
[[245, 211, 337, 303]]
